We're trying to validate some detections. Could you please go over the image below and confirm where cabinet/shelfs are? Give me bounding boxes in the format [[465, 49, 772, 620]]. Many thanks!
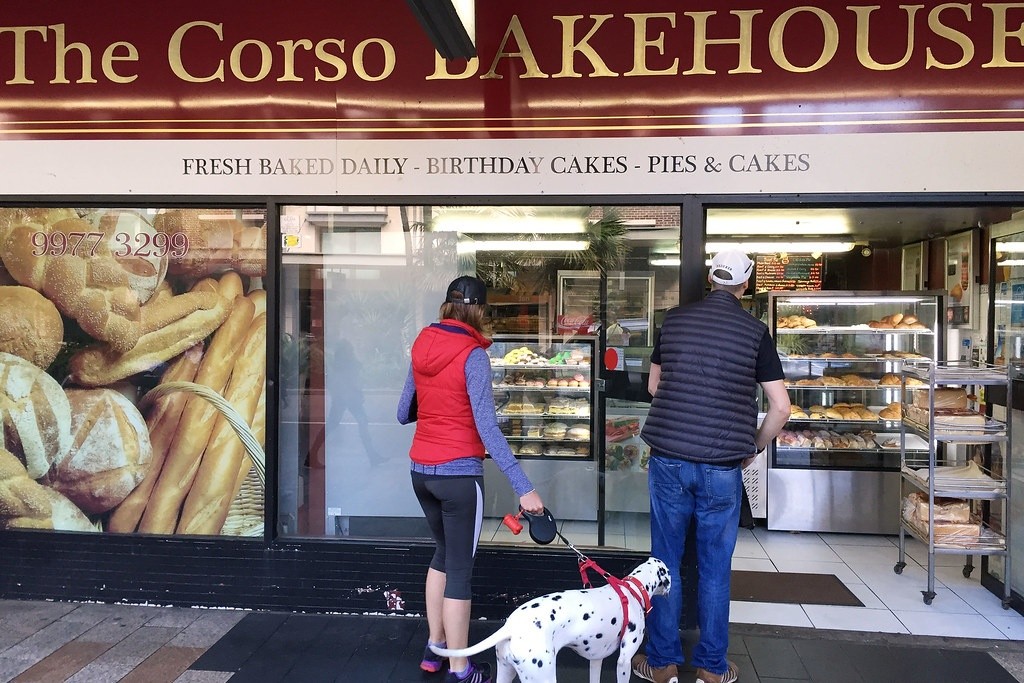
[[764, 290, 949, 534], [485, 335, 598, 520], [606, 414, 767, 515], [894, 357, 1012, 610]]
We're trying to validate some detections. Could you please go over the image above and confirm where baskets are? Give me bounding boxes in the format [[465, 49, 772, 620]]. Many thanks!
[[138, 382, 265, 535]]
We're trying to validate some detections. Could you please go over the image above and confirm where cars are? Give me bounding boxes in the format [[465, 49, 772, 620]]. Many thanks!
[[560, 316, 872, 407]]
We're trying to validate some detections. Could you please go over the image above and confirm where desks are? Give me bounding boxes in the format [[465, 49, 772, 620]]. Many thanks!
[[647, 306, 674, 327]]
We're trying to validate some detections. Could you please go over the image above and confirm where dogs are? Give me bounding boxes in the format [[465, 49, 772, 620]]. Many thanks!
[[428, 557, 672, 683]]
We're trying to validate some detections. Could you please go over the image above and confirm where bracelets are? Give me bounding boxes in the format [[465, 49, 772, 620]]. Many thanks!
[[755, 447, 765, 454]]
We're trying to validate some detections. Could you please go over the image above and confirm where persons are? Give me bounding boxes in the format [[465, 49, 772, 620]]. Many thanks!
[[632, 247, 791, 683], [396, 275, 544, 683]]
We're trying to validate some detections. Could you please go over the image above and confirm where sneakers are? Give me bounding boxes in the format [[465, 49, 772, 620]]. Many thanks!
[[443, 662, 492, 683], [631, 654, 679, 683], [419, 641, 449, 671], [695, 660, 739, 683]]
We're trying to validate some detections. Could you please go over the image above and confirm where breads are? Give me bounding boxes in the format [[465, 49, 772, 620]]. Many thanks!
[[776, 350, 923, 448], [776, 316, 818, 332], [482, 347, 590, 457], [904, 388, 985, 548], [0, 205, 266, 536], [870, 312, 932, 332]]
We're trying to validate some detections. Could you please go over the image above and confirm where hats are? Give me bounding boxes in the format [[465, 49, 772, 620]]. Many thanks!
[[709, 249, 755, 285], [446, 275, 487, 305]]
